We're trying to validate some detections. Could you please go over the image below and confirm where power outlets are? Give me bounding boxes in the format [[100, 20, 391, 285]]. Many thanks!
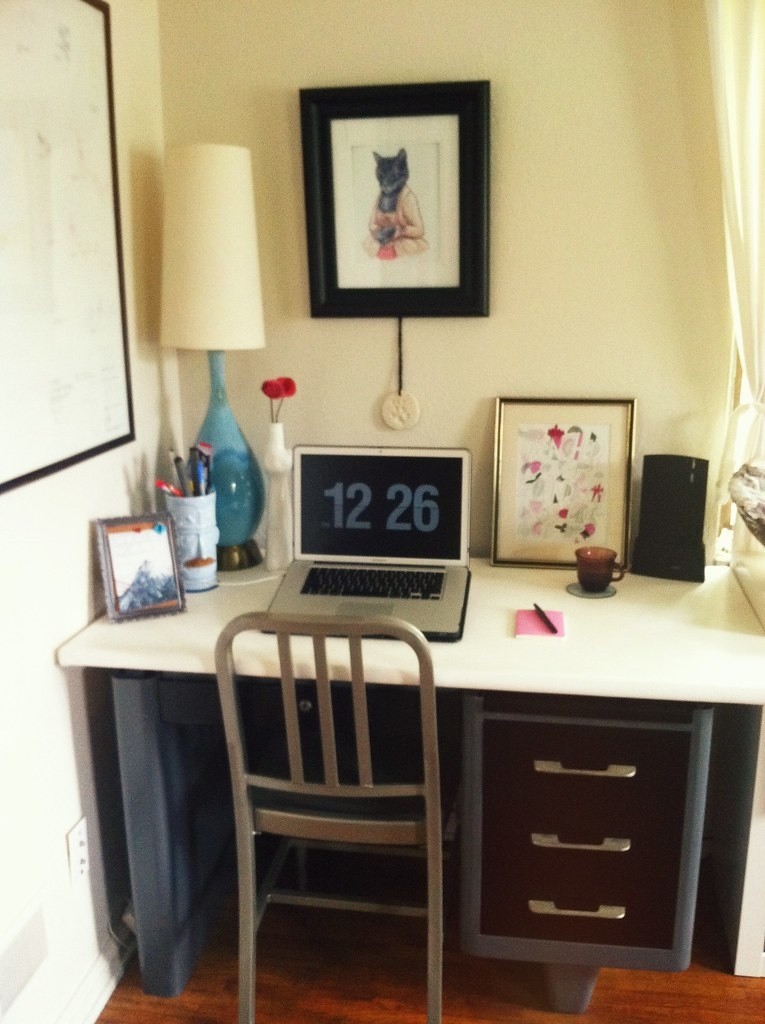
[[67, 817, 96, 875]]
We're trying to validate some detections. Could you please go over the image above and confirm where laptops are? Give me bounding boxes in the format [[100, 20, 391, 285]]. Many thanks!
[[267, 445, 471, 643]]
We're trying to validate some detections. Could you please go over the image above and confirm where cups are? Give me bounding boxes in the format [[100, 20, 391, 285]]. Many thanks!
[[575, 547, 624, 593], [165, 491, 217, 591]]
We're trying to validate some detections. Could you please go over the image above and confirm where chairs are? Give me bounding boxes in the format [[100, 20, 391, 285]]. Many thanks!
[[213, 613, 457, 1023]]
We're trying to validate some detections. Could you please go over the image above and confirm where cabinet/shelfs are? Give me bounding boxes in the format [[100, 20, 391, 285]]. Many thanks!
[[103, 669, 717, 1014]]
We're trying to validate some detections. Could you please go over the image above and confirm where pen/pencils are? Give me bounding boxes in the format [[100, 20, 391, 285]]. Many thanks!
[[534, 603, 557, 633], [155, 446, 210, 497]]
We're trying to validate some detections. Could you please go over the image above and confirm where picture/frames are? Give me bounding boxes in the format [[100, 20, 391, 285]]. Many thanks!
[[300, 79, 491, 319], [95, 514, 186, 621], [489, 398, 634, 569]]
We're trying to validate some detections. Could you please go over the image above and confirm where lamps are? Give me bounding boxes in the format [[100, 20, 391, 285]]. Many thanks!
[[160, 141, 268, 572]]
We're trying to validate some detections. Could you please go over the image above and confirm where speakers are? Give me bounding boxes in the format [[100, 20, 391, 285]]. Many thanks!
[[630, 454, 709, 583]]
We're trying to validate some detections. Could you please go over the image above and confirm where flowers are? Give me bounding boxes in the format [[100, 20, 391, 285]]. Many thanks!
[[262, 377, 297, 424]]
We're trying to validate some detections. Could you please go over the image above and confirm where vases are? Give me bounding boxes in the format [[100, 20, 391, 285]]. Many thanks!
[[261, 422, 295, 572]]
